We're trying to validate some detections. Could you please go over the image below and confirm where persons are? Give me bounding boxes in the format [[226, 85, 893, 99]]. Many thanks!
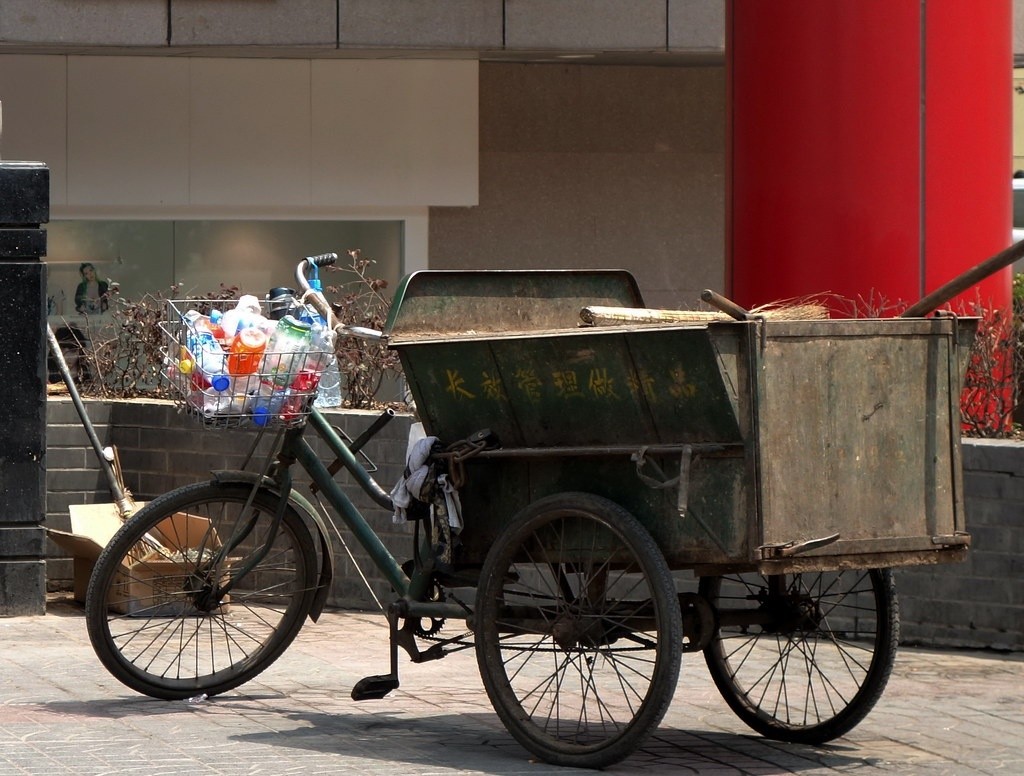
[[46, 321, 98, 397], [73, 261, 112, 317]]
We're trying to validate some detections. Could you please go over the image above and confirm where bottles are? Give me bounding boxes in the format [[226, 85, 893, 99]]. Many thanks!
[[163, 295, 341, 425]]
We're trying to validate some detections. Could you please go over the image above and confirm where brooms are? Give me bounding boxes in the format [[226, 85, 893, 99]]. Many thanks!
[[700, 289, 831, 321], [47, 321, 172, 566]]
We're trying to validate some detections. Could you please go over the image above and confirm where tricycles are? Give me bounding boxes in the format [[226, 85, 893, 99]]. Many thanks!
[[85, 253, 985, 769]]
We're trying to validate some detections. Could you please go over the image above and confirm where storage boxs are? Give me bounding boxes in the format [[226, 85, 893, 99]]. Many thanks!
[[37, 500, 230, 619]]
